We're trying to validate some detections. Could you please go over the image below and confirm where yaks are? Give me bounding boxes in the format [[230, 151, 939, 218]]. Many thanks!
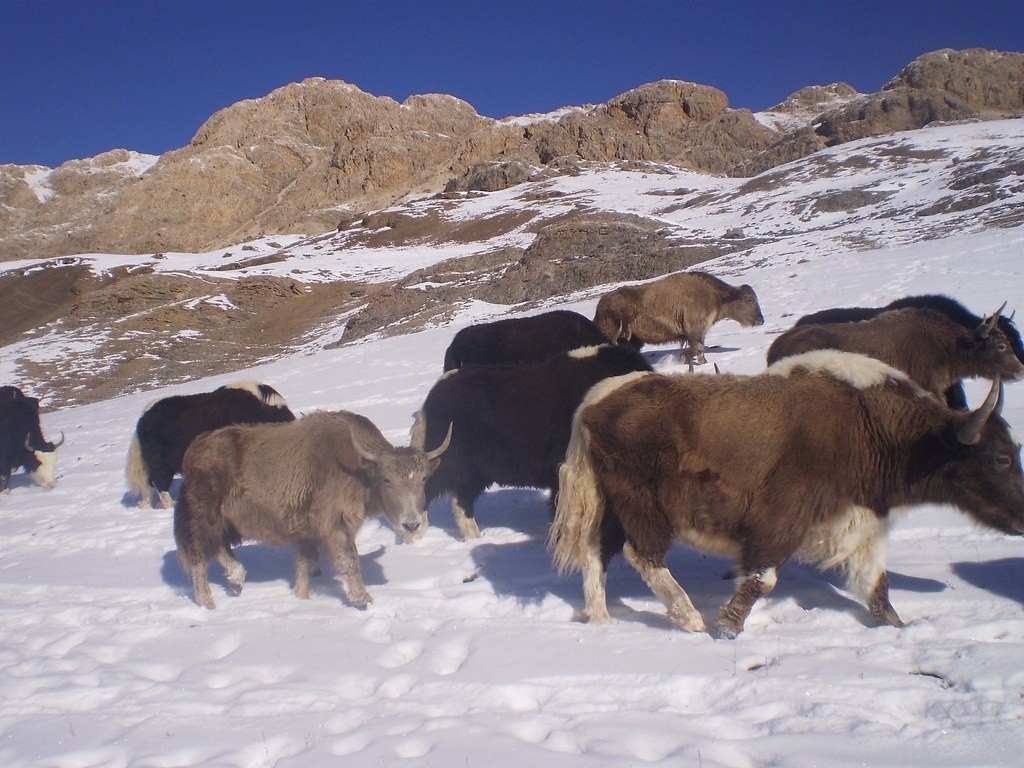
[[0, 384, 67, 490], [123, 381, 453, 613], [407, 270, 1024, 642]]
